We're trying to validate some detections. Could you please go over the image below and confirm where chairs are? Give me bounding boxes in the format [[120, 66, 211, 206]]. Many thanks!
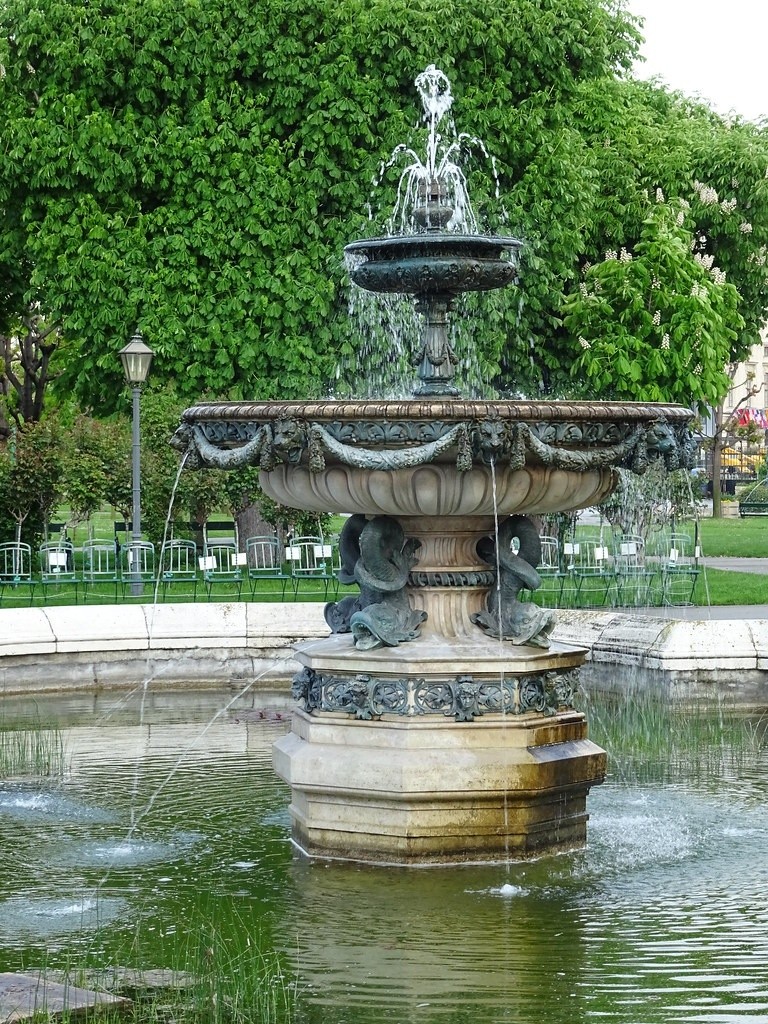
[[82, 539, 121, 604], [0, 541, 39, 607], [521, 536, 568, 608], [161, 539, 201, 603], [329, 534, 344, 602], [644, 533, 701, 605], [203, 540, 246, 602], [289, 537, 332, 602], [40, 541, 81, 605], [602, 534, 656, 608], [246, 536, 290, 602], [557, 536, 625, 607], [121, 541, 160, 603]]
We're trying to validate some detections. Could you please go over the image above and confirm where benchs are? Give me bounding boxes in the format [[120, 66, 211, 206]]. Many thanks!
[[113, 521, 234, 561]]
